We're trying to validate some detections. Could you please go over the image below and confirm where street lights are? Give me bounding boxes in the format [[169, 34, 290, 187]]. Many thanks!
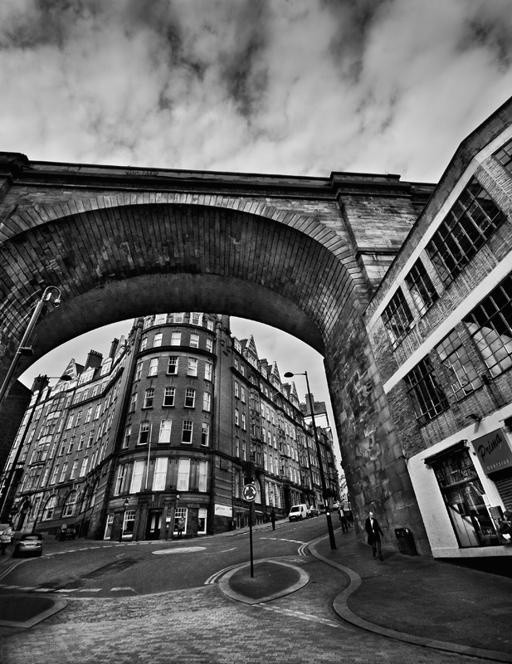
[[0, 286, 62, 408], [283, 369, 341, 555], [0, 374, 73, 511]]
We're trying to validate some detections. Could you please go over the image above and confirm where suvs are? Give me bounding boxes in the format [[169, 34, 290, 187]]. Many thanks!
[[54, 523, 78, 542]]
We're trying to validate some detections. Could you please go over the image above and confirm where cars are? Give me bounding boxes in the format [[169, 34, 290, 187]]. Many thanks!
[[309, 499, 342, 517], [14, 533, 46, 556]]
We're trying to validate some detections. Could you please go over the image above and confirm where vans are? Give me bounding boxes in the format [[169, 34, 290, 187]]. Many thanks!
[[288, 503, 308, 521]]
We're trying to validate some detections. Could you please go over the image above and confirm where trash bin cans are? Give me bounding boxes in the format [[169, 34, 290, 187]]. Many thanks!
[[394, 527, 416, 556]]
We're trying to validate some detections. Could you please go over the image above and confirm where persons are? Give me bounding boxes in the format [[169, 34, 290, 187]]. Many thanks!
[[337, 504, 348, 535], [269, 509, 276, 530], [177, 517, 185, 536], [365, 511, 384, 562]]
